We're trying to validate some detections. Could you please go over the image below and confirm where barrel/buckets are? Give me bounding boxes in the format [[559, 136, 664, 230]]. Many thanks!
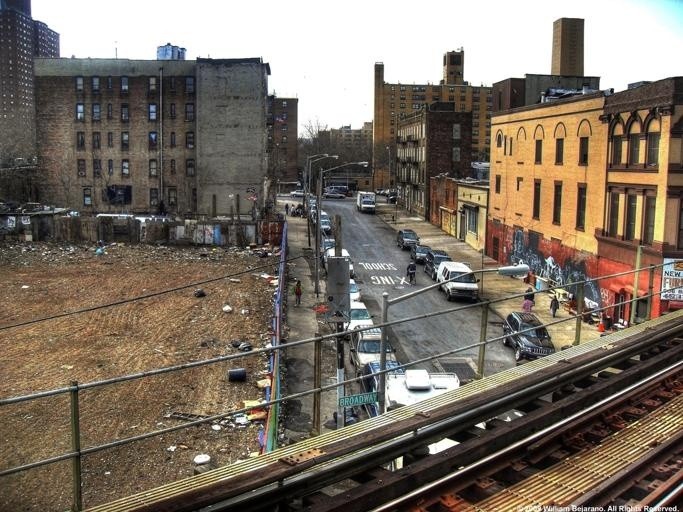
[[598, 323, 604, 332], [228, 367, 247, 383]]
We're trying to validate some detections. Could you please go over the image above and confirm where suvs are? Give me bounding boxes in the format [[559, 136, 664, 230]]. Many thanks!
[[348, 324, 397, 380], [357, 360, 404, 419], [396, 230, 421, 250], [503, 310, 554, 362], [423, 249, 450, 282]]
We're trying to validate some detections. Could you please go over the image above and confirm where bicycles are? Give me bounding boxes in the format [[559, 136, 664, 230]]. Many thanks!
[[407, 270, 417, 285]]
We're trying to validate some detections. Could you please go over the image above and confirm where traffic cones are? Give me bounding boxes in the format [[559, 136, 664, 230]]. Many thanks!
[[598, 320, 605, 332]]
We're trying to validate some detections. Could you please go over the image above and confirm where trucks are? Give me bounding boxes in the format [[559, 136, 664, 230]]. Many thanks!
[[356, 191, 378, 214], [383, 368, 490, 473]]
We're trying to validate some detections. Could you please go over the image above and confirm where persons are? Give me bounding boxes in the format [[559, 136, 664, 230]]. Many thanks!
[[550, 295, 559, 318], [291, 279, 301, 304], [522, 296, 533, 312], [285, 203, 288, 214], [290, 203, 295, 215], [406, 260, 415, 281], [525, 286, 534, 301]]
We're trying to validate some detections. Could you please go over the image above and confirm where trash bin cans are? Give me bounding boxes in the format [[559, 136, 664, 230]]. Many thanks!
[[602, 318, 610, 330]]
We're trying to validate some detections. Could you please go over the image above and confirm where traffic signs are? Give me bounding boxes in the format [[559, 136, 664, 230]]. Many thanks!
[[338, 391, 379, 409]]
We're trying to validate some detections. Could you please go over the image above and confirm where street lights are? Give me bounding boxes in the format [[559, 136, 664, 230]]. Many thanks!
[[375, 264, 531, 416], [313, 161, 369, 295], [308, 155, 340, 227], [386, 146, 392, 194], [306, 153, 329, 212]]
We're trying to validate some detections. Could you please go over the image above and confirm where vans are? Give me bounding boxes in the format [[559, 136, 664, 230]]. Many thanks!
[[324, 249, 356, 278], [435, 259, 480, 303], [323, 185, 349, 192]]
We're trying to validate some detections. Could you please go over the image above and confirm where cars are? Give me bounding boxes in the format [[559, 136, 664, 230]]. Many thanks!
[[309, 200, 331, 234], [410, 243, 431, 263], [350, 278, 362, 301], [376, 187, 397, 204], [320, 239, 336, 254], [323, 192, 345, 199], [343, 301, 375, 340], [289, 189, 313, 198]]
